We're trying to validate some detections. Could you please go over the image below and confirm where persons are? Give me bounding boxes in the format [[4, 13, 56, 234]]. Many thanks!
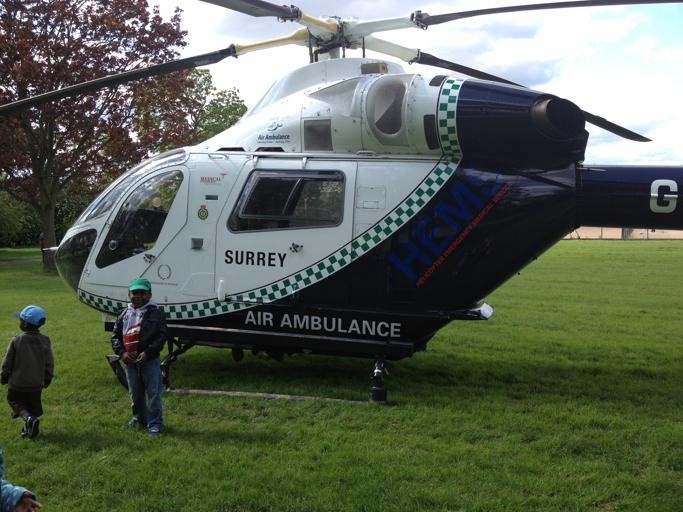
[[40, 232, 44, 264], [0, 450, 42, 511], [0, 305, 54, 437], [110, 277, 168, 434]]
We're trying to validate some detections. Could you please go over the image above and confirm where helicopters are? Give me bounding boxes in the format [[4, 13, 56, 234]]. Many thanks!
[[1, 0, 683, 403]]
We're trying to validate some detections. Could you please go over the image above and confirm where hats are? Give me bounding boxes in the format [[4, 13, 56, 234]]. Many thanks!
[[15, 304, 47, 327], [129, 278, 151, 291]]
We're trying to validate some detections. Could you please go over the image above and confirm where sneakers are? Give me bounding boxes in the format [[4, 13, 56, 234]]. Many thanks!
[[122, 418, 146, 430], [21, 416, 39, 438], [149, 423, 164, 434]]
